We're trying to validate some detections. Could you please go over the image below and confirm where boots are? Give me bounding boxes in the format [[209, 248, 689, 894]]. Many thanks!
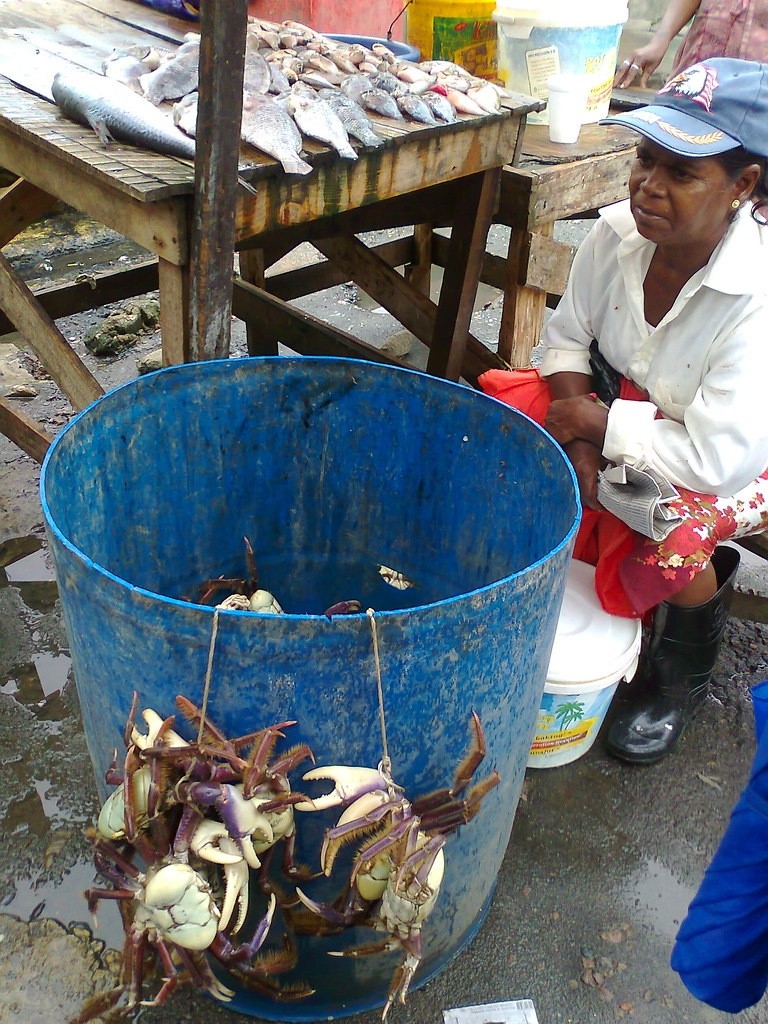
[[604, 548, 741, 764]]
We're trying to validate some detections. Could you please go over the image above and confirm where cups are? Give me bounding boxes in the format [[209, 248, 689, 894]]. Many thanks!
[[546, 77, 589, 144]]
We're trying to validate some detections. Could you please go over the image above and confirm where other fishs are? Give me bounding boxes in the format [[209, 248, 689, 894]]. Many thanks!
[[50, 19, 512, 197]]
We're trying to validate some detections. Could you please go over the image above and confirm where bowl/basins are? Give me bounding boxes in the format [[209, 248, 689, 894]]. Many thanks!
[[318, 33, 421, 64]]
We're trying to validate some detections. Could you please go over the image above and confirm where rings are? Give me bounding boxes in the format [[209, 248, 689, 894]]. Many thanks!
[[631, 64, 639, 70], [623, 59, 630, 66]]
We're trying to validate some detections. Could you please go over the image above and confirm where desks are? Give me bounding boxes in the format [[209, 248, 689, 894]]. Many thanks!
[[0, 0, 547, 467], [242, 103, 646, 397]]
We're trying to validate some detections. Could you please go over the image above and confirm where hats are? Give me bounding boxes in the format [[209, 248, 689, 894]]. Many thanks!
[[599, 57, 766, 162]]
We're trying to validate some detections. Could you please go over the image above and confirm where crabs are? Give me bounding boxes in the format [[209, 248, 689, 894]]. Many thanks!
[[65, 692, 502, 1024], [181, 536, 361, 623]]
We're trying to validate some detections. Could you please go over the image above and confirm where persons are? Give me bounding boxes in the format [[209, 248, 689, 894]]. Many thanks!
[[530, 56, 768, 765], [612, 0, 768, 89]]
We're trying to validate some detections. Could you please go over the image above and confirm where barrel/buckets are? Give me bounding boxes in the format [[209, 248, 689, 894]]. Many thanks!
[[491, 0, 629, 125], [388, 1, 497, 86], [526, 557, 641, 769], [40, 356, 583, 1018]]
